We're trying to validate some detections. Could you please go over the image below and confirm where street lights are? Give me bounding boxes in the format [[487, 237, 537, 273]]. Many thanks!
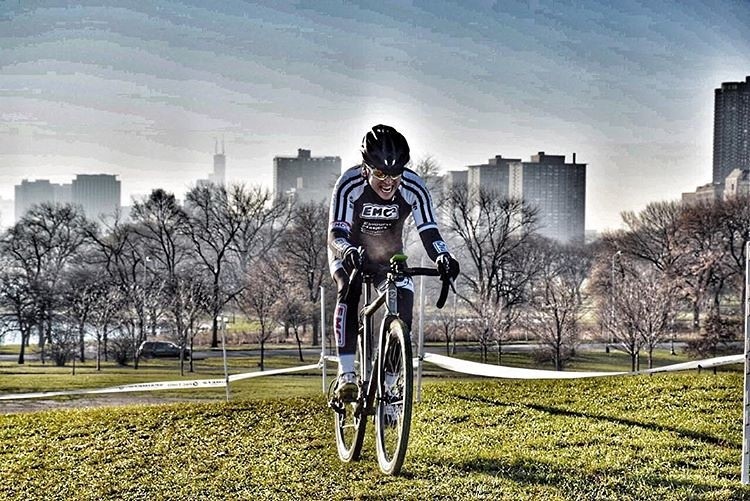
[[611, 251, 625, 340]]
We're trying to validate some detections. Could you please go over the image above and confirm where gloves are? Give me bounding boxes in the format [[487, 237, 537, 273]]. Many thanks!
[[436, 254, 460, 284], [341, 248, 367, 278]]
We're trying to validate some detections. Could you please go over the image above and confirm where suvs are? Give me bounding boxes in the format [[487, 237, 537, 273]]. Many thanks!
[[136, 340, 192, 361]]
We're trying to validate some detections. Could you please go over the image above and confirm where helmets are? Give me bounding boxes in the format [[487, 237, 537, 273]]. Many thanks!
[[361, 124, 410, 174]]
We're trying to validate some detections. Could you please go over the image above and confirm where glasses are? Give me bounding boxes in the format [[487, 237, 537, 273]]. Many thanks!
[[362, 163, 402, 180]]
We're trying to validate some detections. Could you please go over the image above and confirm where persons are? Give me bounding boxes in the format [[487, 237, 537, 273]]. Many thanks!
[[328, 124, 460, 429]]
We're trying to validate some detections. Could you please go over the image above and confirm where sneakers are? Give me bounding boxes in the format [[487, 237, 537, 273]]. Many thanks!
[[383, 404, 399, 427], [334, 372, 358, 399]]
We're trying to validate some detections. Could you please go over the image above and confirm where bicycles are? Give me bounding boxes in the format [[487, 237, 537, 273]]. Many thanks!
[[327, 251, 458, 481]]
